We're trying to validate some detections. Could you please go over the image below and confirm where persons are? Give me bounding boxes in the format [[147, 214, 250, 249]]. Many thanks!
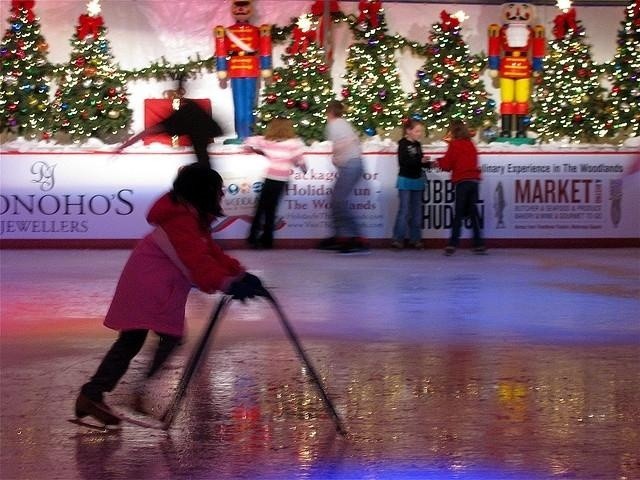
[[67, 163, 263, 432], [430, 118, 488, 254], [387, 118, 431, 250], [212, 1, 274, 144], [488, 2, 543, 144], [241, 117, 308, 248], [313, 100, 374, 256], [493, 182, 507, 230]]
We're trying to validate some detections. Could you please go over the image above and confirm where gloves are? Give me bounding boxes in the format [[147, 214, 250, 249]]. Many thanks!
[[228, 272, 269, 301]]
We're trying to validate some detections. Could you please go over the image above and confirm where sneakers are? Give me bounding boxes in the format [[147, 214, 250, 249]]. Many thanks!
[[245, 232, 271, 245], [154, 329, 184, 344], [317, 236, 366, 250], [443, 245, 487, 255], [75, 387, 122, 425], [392, 238, 424, 250]]
